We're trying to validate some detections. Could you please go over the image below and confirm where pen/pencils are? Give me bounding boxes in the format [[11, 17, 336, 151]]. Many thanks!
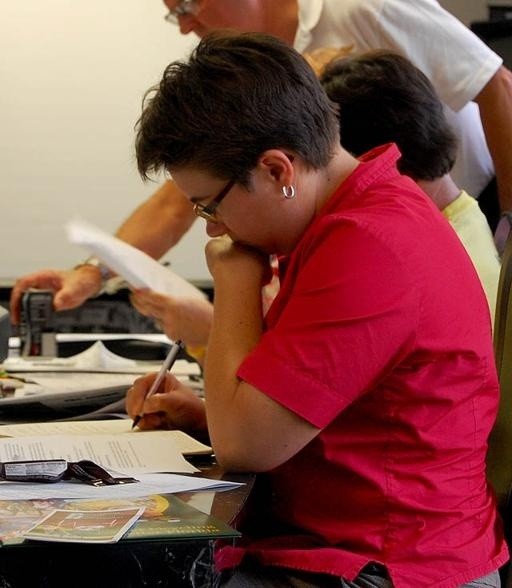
[[130, 339, 183, 431]]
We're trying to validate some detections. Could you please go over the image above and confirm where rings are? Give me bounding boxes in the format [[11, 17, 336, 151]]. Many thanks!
[[500, 208, 512, 223], [131, 387, 135, 390]]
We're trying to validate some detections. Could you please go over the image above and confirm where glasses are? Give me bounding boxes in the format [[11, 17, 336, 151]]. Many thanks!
[[193, 178, 235, 224], [166, 0, 205, 26]]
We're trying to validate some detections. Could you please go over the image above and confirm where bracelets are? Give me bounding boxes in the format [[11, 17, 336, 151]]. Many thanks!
[[75, 259, 108, 300]]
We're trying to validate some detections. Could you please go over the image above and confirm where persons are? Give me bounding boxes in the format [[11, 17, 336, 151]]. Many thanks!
[[123, 47, 512, 508], [7, 0, 512, 334], [125, 29, 512, 588]]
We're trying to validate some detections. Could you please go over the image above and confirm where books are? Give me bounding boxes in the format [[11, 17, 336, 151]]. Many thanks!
[[1, 338, 246, 544]]
